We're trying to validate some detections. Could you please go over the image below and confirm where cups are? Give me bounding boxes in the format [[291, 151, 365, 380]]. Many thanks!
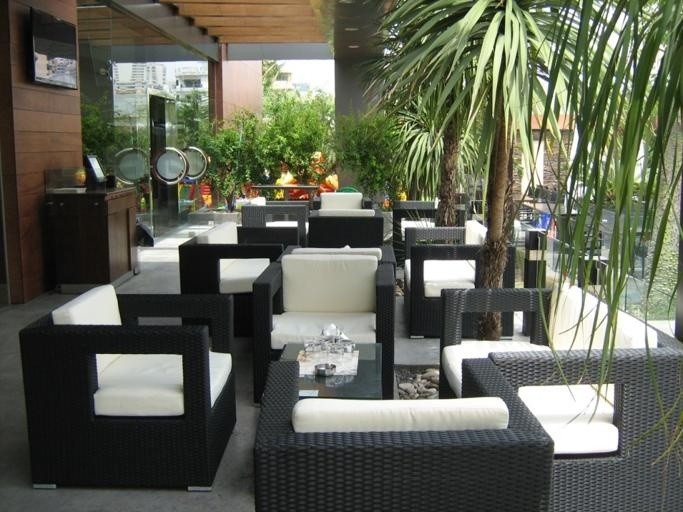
[[303, 334, 355, 370]]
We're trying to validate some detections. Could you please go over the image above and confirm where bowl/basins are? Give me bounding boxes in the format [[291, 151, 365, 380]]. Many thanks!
[[314, 364, 335, 376], [314, 375, 335, 384]]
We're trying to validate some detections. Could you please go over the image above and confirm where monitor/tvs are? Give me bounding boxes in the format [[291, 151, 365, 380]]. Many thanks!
[[30, 7, 78, 90]]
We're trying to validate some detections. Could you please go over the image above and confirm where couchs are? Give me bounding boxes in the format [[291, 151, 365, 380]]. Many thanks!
[[21, 187, 683, 510]]
